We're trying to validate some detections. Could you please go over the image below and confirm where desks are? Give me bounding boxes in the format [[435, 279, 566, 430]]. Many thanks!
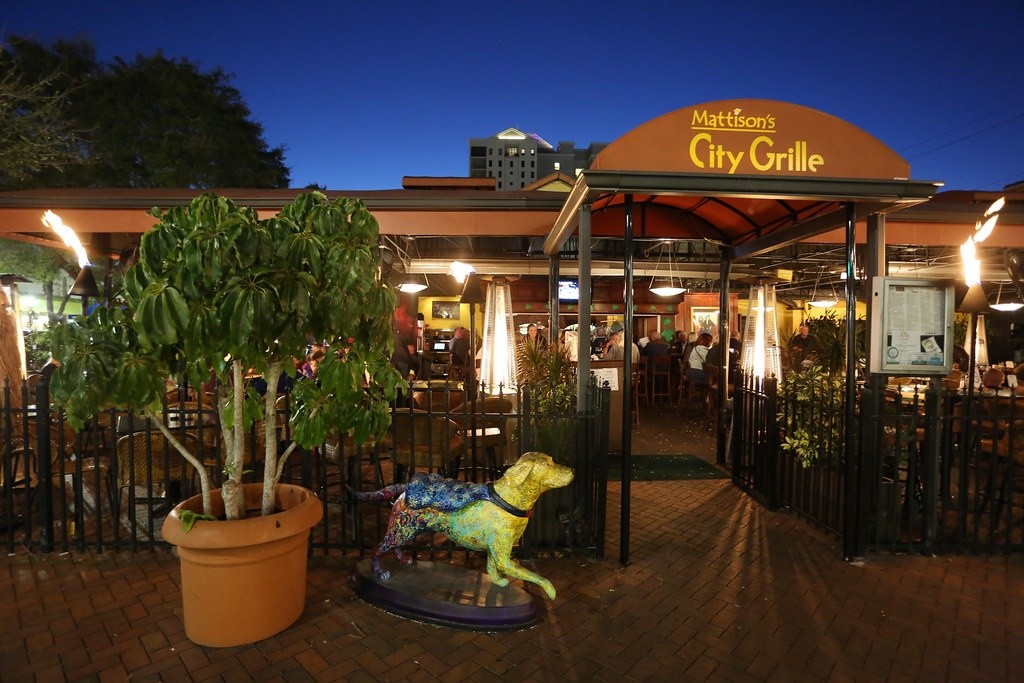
[[882, 384, 1024, 509]]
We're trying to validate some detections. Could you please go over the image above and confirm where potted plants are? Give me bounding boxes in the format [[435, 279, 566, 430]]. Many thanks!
[[47, 189, 407, 648]]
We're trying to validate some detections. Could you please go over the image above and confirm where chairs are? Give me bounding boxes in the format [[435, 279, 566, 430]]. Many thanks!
[[0, 337, 737, 547], [840, 364, 1024, 540]]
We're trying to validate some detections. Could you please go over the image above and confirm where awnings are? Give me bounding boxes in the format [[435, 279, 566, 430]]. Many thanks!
[[541, 100, 945, 562]]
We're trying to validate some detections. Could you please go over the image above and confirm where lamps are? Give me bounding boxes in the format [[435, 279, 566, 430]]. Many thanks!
[[806, 266, 839, 308], [989, 281, 1024, 312], [394, 235, 429, 294], [648, 241, 686, 297]]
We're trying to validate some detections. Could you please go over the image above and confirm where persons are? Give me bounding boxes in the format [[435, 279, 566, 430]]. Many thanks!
[[254, 344, 326, 397], [41, 355, 61, 379], [515, 324, 569, 353], [638, 331, 668, 376], [449, 326, 471, 364], [789, 323, 819, 369], [407, 344, 431, 380], [592, 323, 639, 386], [673, 330, 743, 385]]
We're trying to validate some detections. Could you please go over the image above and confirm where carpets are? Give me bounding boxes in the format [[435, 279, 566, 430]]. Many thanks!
[[605, 454, 732, 481]]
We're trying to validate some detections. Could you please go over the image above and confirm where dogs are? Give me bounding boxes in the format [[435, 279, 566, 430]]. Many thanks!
[[343, 452, 575, 600]]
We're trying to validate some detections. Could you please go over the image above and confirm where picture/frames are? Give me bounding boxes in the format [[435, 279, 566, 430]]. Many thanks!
[[690, 306, 720, 338]]
